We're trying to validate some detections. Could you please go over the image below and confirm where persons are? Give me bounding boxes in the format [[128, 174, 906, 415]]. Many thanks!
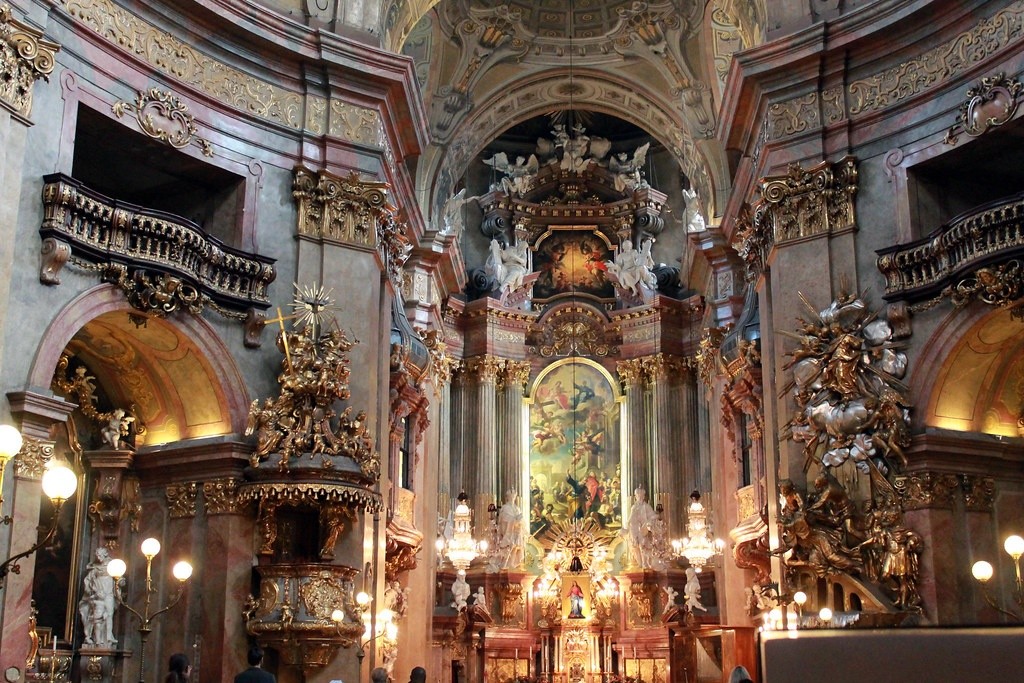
[[604, 240, 653, 297], [163, 653, 192, 683], [408, 667, 426, 683], [256, 326, 367, 474], [478, 587, 486, 607], [501, 241, 529, 290], [729, 665, 753, 683], [371, 667, 388, 683], [71, 367, 98, 402], [451, 569, 470, 609], [84, 548, 119, 645], [662, 586, 675, 615], [100, 409, 125, 451], [618, 152, 627, 165], [320, 517, 344, 555], [619, 488, 655, 570], [256, 494, 284, 552], [444, 195, 479, 235], [384, 581, 410, 672], [681, 188, 704, 233], [685, 568, 707, 612], [555, 123, 582, 148], [501, 156, 530, 198], [234, 648, 277, 683], [768, 322, 917, 609], [498, 490, 524, 568]]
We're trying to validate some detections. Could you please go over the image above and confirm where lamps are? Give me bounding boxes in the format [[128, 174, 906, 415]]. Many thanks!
[[0, 423, 78, 578], [971, 535, 1024, 616], [435, 1, 729, 603], [333, 592, 392, 650], [792, 592, 834, 628], [106, 535, 194, 623]]
